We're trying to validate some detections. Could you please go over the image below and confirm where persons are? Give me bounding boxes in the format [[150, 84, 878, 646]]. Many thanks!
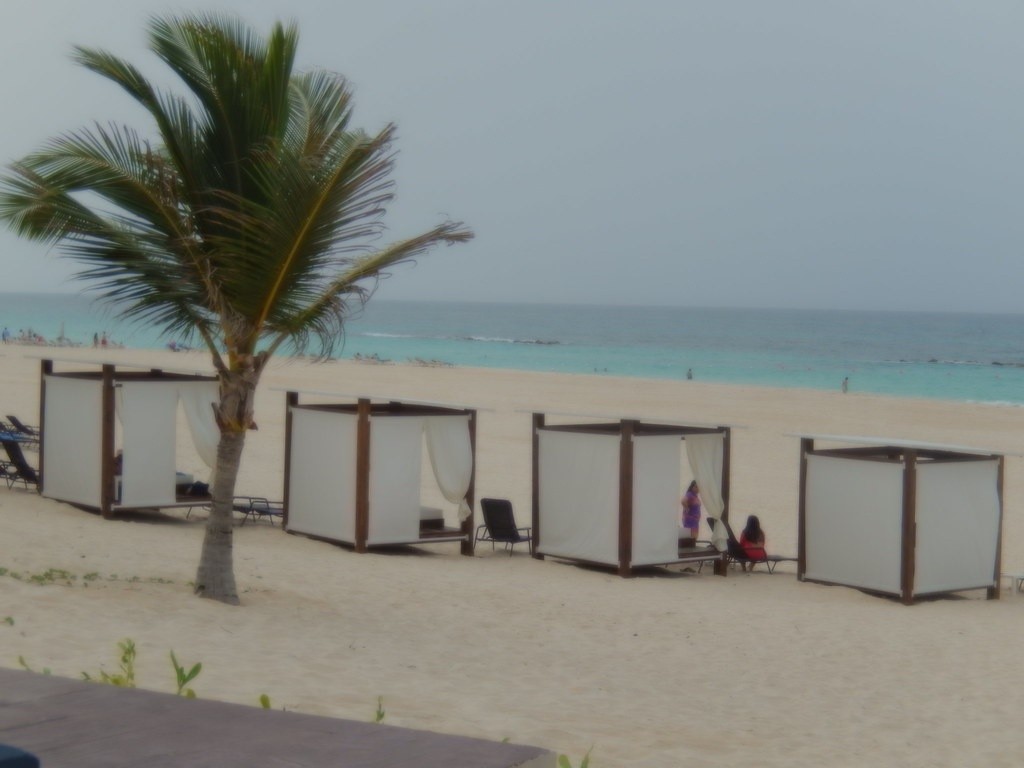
[[843, 376, 849, 391], [681, 479, 701, 541], [687, 368, 692, 379], [739, 515, 767, 571], [93, 331, 108, 348], [2, 326, 46, 345]]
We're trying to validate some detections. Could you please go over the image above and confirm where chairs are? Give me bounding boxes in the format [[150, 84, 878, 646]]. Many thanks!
[[725, 539, 798, 573], [232, 495, 283, 526], [474, 498, 532, 557], [186, 481, 211, 518], [0, 414, 40, 491]]
